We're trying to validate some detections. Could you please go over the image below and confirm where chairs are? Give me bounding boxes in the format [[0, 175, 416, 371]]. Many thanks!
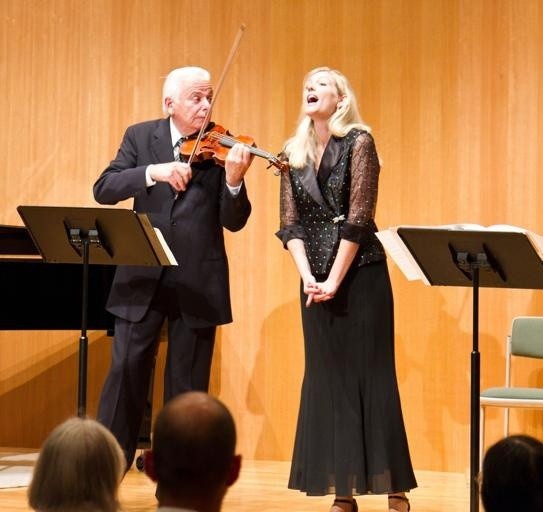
[[478, 314, 543, 470]]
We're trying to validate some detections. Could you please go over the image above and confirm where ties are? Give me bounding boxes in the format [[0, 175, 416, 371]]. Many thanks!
[[176, 137, 188, 163]]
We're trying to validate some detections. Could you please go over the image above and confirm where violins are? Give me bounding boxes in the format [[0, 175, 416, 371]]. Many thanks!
[[178, 124, 291, 176]]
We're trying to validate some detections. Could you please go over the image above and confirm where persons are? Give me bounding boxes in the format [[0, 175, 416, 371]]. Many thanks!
[[25, 417, 129, 512], [481, 434, 542, 511], [94, 65, 255, 474], [141, 391, 245, 512], [271, 65, 421, 512]]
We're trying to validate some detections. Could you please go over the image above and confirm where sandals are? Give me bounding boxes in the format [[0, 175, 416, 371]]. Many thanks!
[[335, 498, 358, 512], [388, 496, 410, 512]]
